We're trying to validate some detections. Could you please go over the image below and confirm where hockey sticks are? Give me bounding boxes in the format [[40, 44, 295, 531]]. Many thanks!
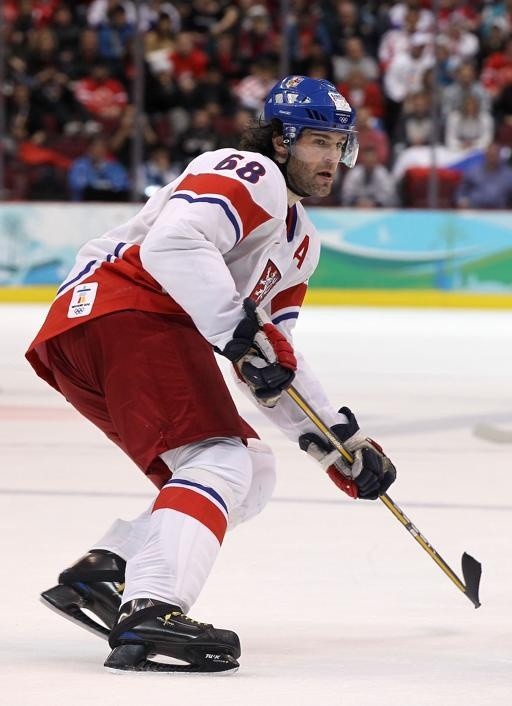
[[286, 386, 481, 612]]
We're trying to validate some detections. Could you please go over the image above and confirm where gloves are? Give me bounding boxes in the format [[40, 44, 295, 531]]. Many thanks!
[[216, 294, 296, 399], [299, 404, 398, 502]]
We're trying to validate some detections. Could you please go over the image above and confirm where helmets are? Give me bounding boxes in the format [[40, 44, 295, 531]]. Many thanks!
[[260, 74, 361, 172]]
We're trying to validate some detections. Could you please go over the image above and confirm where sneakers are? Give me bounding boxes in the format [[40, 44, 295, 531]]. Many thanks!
[[106, 595, 242, 661], [55, 549, 140, 628]]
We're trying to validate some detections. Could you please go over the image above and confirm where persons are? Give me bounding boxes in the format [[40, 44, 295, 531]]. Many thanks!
[[24, 75, 398, 674]]
